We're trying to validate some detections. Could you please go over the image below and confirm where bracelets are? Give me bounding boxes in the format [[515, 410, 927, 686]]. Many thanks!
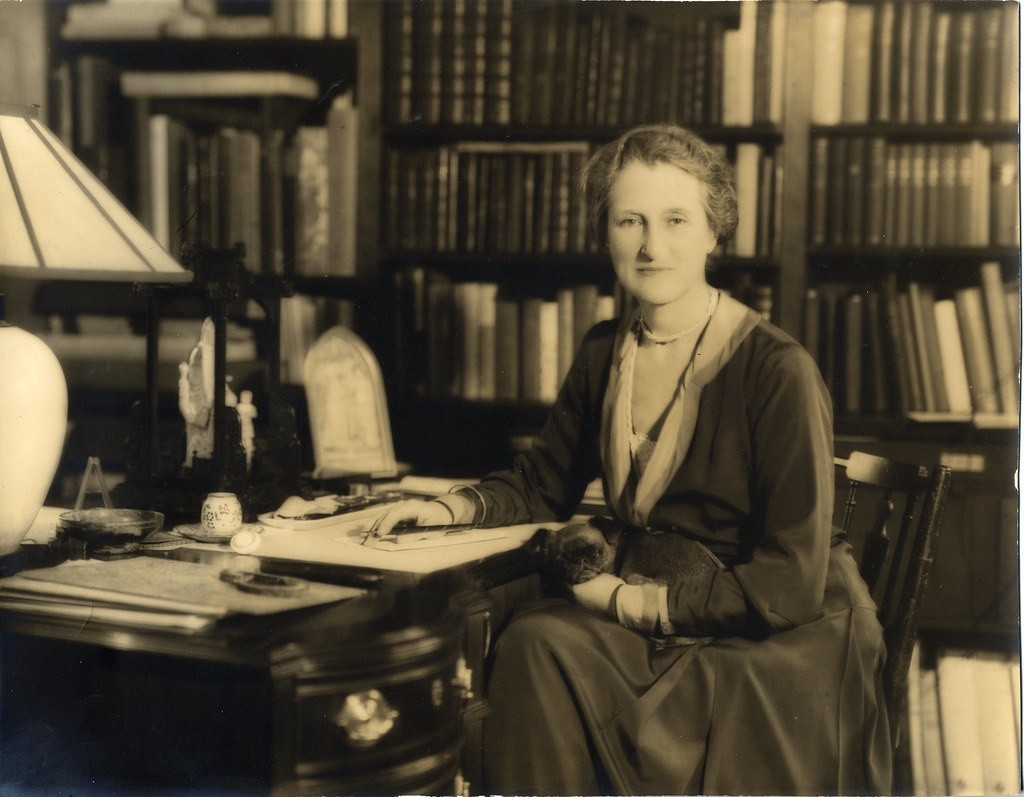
[[430, 495, 463, 526], [448, 482, 486, 526], [610, 582, 674, 637]]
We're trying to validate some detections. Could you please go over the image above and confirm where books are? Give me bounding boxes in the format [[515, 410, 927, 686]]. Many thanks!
[[43, 1, 1021, 427]]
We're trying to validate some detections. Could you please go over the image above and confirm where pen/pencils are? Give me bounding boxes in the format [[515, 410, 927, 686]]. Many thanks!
[[361, 521, 488, 535]]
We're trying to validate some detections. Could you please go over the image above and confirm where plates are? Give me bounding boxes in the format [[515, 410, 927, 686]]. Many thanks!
[[257, 495, 405, 531]]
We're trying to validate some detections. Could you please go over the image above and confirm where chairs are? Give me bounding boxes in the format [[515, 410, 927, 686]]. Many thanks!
[[834, 451, 950, 796]]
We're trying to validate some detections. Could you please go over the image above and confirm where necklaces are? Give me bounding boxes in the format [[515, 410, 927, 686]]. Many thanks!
[[638, 286, 718, 343]]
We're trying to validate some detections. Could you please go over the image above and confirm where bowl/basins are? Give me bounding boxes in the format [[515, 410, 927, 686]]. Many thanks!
[[58, 507, 166, 554]]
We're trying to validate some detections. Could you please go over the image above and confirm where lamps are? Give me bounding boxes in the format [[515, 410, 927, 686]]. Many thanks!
[[0, 104, 194, 577]]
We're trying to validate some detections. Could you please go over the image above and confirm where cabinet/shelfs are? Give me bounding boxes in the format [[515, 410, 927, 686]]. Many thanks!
[[0, 503, 611, 797], [353, 0, 1022, 656]]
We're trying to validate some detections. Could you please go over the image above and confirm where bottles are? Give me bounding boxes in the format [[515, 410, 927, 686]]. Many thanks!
[[200, 492, 243, 537]]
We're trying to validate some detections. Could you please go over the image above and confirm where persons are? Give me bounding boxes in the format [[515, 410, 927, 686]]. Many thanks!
[[363, 124, 893, 797]]
